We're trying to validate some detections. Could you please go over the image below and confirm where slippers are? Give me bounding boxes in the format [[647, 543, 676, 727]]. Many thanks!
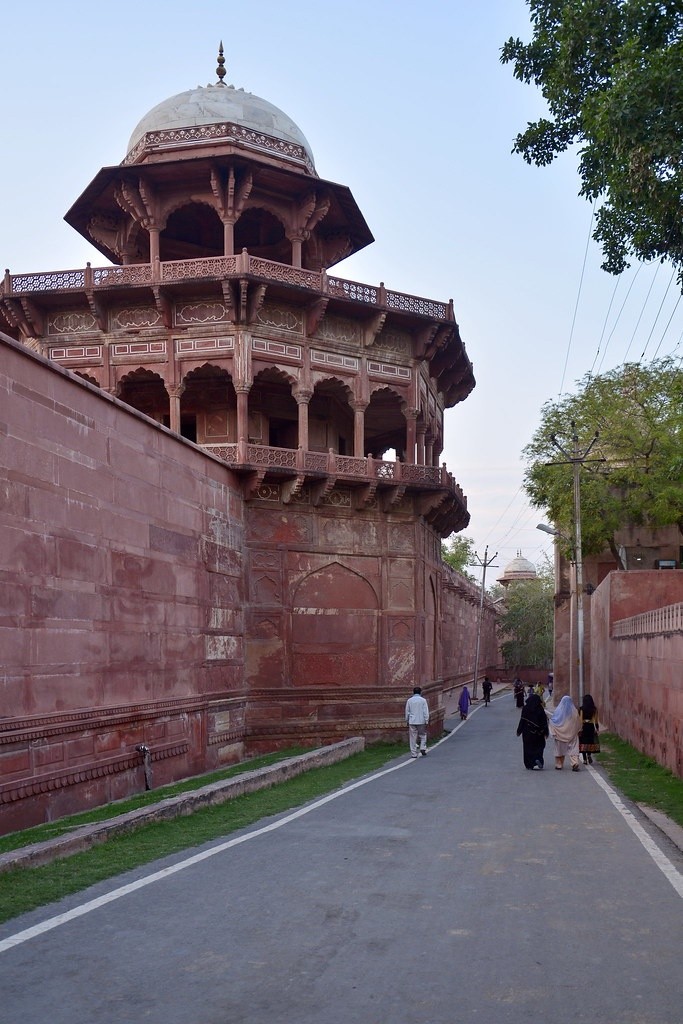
[[572, 766, 579, 771], [555, 766, 561, 770]]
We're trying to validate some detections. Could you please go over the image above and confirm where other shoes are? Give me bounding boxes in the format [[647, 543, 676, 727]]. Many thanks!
[[536, 760, 542, 767], [587, 755, 593, 764], [421, 749, 426, 755], [583, 760, 587, 764]]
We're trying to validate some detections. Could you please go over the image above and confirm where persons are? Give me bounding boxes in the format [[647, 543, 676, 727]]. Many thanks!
[[481, 677, 493, 707], [577, 694, 601, 764], [511, 678, 547, 708], [548, 695, 582, 772], [547, 677, 553, 696], [516, 694, 549, 770], [457, 686, 472, 720], [405, 687, 429, 759]]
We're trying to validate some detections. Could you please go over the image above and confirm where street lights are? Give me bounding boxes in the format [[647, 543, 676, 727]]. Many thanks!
[[536, 523, 585, 710]]
